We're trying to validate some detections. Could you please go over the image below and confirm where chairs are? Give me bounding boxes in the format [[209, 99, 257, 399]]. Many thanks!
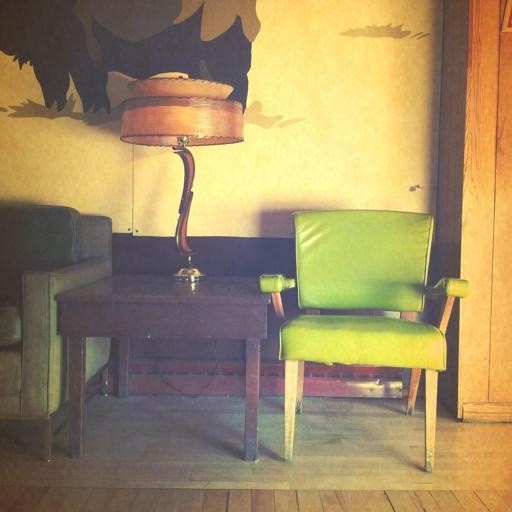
[[259, 209, 469, 472]]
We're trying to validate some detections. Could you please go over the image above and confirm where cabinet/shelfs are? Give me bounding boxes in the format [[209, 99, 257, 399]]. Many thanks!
[[438, 0, 512, 424]]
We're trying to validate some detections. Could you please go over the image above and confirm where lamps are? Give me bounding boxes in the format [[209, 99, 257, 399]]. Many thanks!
[[119, 76, 244, 283]]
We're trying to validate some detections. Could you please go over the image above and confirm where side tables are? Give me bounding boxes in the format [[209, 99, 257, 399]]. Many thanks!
[[54, 274, 270, 462]]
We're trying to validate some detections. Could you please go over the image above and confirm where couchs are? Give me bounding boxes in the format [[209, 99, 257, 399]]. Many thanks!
[[0, 203, 112, 463]]
